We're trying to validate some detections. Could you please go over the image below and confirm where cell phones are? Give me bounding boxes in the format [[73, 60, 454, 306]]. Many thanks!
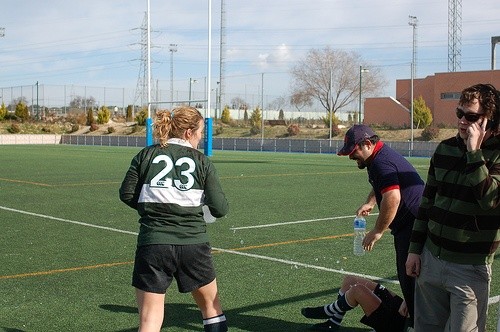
[[479, 119, 495, 130]]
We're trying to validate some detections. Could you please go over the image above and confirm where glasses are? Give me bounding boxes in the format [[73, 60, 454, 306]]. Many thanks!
[[457, 109, 483, 122]]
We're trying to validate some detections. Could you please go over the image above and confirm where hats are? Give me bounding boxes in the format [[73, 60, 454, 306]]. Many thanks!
[[337, 124, 374, 156]]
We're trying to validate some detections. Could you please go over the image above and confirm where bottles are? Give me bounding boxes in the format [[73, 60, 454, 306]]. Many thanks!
[[353, 213, 367, 257], [202, 193, 216, 223]]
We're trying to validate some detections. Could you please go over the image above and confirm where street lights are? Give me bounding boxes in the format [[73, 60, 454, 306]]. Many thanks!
[[407, 15, 418, 151], [188, 78, 197, 106], [359, 66, 371, 124], [35, 80, 39, 117], [168, 43, 178, 101]]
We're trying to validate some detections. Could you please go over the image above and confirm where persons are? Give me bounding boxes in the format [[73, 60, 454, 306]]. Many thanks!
[[118, 105, 229, 332], [300, 274, 415, 332], [406, 84, 500, 332], [337, 123, 428, 329]]
